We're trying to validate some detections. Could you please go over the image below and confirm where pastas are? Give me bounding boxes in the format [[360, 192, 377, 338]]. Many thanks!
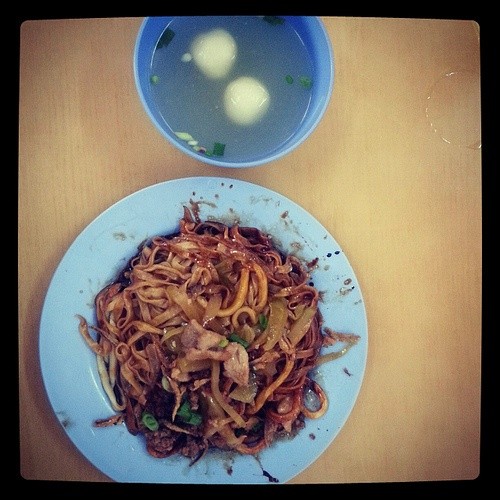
[[77, 205, 359, 468]]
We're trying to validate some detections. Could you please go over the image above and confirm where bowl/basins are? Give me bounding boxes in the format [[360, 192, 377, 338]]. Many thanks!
[[133, 16, 334, 168]]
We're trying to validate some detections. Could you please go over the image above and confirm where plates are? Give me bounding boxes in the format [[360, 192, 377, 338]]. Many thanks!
[[39, 176, 368, 484]]
